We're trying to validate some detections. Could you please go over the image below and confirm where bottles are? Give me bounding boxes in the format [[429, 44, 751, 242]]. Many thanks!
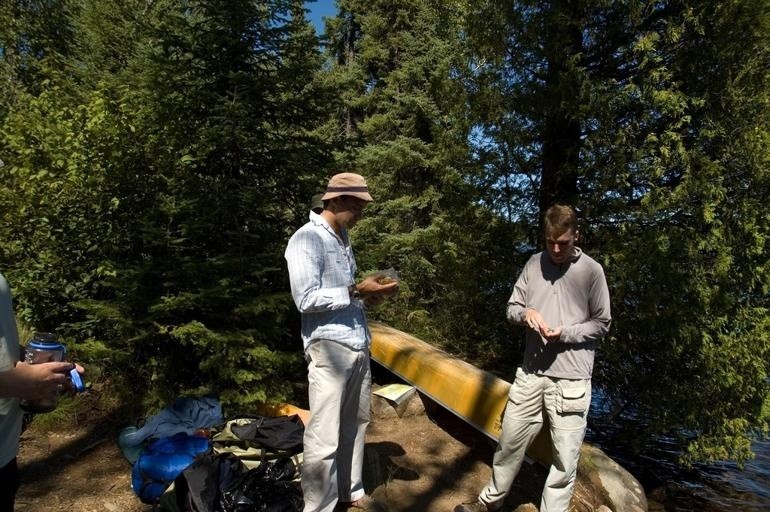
[[19, 330, 66, 414]]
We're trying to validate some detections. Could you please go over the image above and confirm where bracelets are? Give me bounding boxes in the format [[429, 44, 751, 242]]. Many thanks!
[[351, 283, 360, 301]]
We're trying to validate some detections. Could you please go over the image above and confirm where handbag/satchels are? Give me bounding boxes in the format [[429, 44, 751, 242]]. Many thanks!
[[119, 394, 305, 512]]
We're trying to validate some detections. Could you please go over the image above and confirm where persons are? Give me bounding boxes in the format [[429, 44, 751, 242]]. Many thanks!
[[0, 272, 87, 512], [283, 172, 403, 512], [453, 203, 613, 512]]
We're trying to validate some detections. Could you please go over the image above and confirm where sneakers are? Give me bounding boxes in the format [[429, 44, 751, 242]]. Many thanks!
[[454, 500, 488, 512], [350, 495, 388, 511]]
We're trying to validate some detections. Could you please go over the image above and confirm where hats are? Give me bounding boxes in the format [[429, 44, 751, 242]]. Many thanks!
[[321, 173, 374, 203]]
